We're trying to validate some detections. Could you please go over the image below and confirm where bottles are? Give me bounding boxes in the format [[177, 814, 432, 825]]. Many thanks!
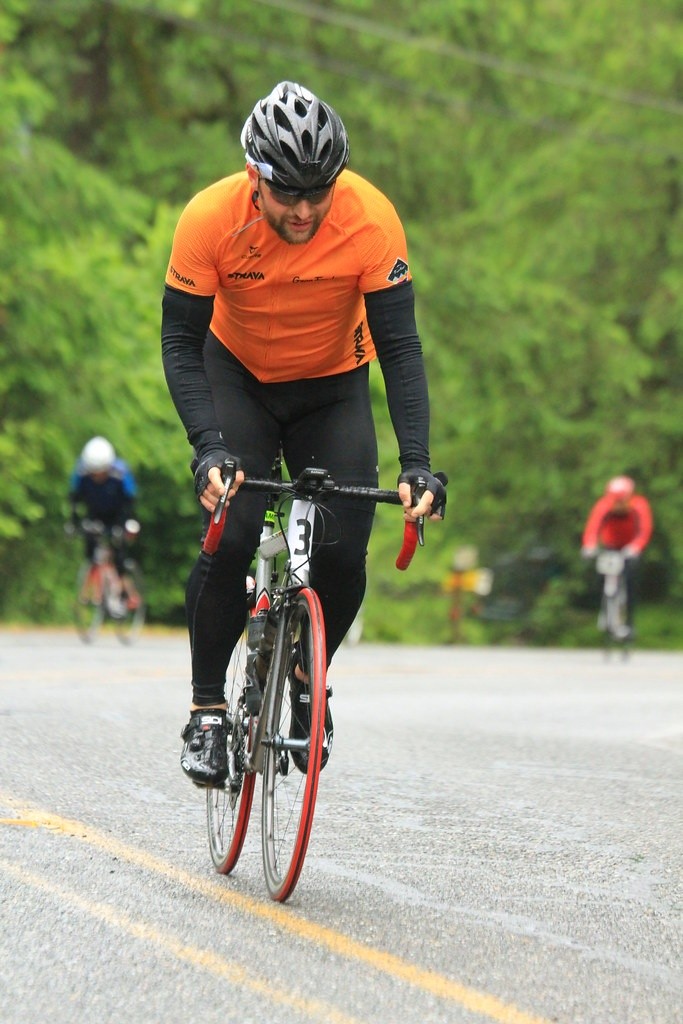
[[254, 587, 284, 683], [245, 608, 267, 717]]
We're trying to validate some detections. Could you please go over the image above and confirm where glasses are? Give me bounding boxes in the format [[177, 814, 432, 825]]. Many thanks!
[[269, 187, 332, 207]]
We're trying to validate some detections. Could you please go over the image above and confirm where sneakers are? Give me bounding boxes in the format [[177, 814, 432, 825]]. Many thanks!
[[181, 708, 228, 786], [287, 652, 334, 774]]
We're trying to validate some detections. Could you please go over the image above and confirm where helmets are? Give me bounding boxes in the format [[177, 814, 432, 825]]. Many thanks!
[[240, 81, 350, 190], [80, 437, 114, 475], [608, 476, 635, 501]]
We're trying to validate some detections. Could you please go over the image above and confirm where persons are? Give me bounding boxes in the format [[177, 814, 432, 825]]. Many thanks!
[[159, 78, 450, 789], [576, 472, 654, 639], [69, 437, 146, 609]]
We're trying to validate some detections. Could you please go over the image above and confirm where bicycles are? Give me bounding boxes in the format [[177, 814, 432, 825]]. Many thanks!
[[183, 456, 428, 904], [64, 517, 147, 646], [585, 548, 631, 643]]
